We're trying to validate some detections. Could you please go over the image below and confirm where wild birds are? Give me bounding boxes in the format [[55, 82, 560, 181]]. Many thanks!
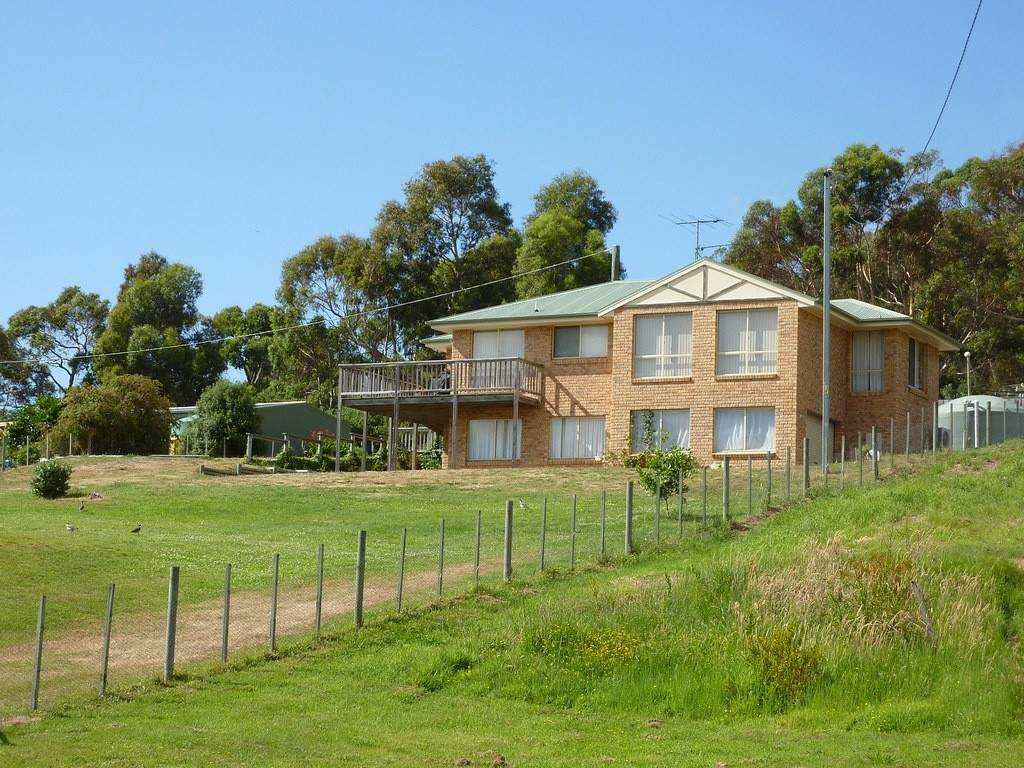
[[518, 498, 527, 511], [78, 500, 85, 511], [91, 492, 103, 500], [65, 522, 75, 533], [130, 524, 142, 536]]
